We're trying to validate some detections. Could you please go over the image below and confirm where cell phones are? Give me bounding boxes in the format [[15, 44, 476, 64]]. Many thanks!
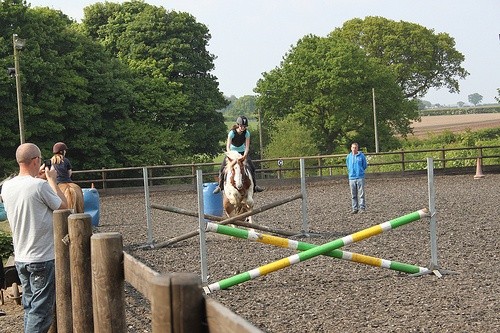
[[43, 160, 51, 171]]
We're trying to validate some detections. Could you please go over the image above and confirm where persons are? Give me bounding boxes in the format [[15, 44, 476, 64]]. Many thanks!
[[346, 142, 368, 214], [48, 142, 73, 184], [213, 116, 263, 194], [1, 143, 68, 333]]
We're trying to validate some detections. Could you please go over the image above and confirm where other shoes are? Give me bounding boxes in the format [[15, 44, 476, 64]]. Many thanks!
[[360, 208, 366, 214], [351, 209, 358, 214]]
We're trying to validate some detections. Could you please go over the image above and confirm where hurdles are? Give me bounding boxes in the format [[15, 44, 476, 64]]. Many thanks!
[[197, 159, 460, 295], [143, 159, 321, 250]]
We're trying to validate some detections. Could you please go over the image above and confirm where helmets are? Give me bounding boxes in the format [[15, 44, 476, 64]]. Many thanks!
[[53, 142, 68, 153], [236, 115, 248, 127]]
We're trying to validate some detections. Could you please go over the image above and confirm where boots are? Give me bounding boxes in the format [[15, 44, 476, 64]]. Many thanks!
[[213, 172, 224, 194], [251, 171, 265, 192]]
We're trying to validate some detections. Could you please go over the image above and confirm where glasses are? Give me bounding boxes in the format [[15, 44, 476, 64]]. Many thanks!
[[31, 155, 44, 162]]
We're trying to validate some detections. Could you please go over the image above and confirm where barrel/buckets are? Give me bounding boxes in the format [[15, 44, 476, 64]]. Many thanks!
[[202, 181, 224, 216], [82, 188, 100, 226]]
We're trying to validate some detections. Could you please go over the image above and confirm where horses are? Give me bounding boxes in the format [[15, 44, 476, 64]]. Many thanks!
[[222, 152, 255, 237], [36, 170, 85, 214]]
